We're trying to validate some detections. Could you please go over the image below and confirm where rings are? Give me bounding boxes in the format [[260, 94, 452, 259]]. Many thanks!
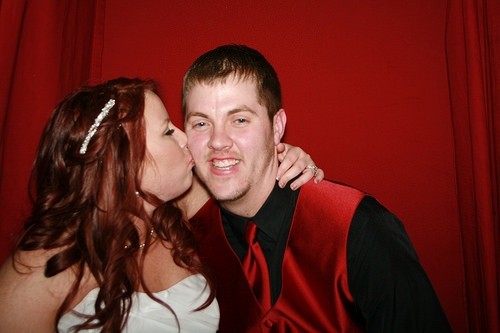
[[305, 166, 319, 179]]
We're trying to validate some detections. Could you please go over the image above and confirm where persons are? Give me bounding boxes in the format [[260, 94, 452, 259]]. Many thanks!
[[164, 45, 452, 333], [0, 77, 221, 333]]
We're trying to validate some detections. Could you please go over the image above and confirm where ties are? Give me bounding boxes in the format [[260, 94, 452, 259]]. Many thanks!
[[240, 221, 272, 315]]
